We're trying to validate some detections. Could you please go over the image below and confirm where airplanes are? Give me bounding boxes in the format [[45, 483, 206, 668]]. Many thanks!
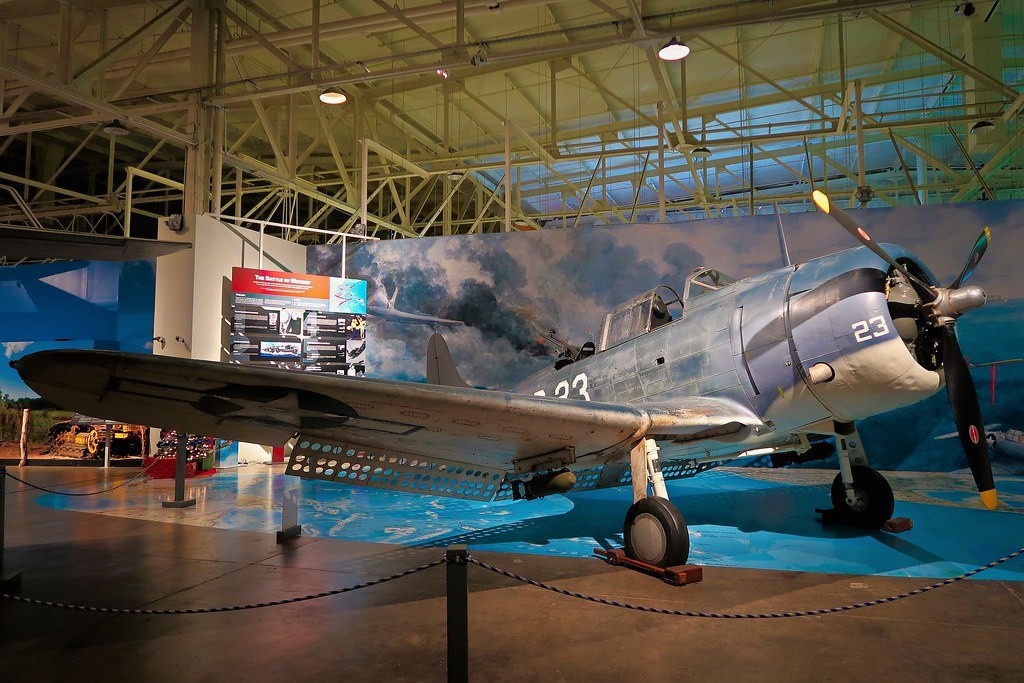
[[10, 188, 996, 566], [933, 423, 1024, 476]]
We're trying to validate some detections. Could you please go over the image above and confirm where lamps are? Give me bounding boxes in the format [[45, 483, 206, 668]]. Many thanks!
[[971, 120, 996, 134], [658, 37, 690, 61], [448, 173, 463, 180], [103, 118, 130, 136], [690, 148, 711, 159], [318, 86, 347, 105], [276, 190, 294, 197]]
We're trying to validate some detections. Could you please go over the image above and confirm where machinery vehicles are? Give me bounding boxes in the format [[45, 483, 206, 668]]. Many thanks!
[[41, 412, 144, 460]]
